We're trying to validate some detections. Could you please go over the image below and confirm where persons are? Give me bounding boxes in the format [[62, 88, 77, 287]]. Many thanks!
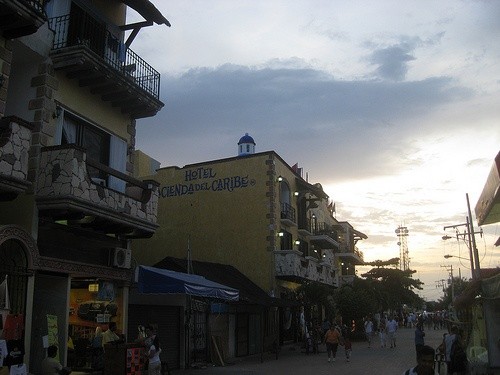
[[379, 319, 387, 348], [363, 318, 374, 348], [396, 311, 448, 328], [61, 366, 73, 375], [144, 325, 157, 344], [440, 326, 469, 375], [401, 346, 440, 375], [387, 316, 398, 349], [39, 346, 63, 375], [146, 336, 163, 375], [414, 322, 426, 359], [341, 336, 353, 362], [102, 322, 121, 353], [322, 318, 342, 361]]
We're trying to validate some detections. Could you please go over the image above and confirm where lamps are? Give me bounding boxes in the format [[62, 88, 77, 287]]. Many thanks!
[[294, 190, 300, 197], [322, 252, 326, 259], [52, 105, 63, 119], [295, 239, 301, 246], [278, 230, 285, 239], [277, 175, 282, 183]]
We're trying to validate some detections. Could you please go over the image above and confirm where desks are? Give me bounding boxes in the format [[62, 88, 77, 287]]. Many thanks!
[[103, 342, 145, 375]]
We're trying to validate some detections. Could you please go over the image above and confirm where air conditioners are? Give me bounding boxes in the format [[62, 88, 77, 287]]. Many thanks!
[[108, 246, 131, 269]]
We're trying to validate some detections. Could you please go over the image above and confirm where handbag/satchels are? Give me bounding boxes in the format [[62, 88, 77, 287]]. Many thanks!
[[438, 335, 446, 353]]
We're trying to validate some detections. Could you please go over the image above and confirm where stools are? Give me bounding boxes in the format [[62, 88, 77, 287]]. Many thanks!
[[161, 361, 169, 375]]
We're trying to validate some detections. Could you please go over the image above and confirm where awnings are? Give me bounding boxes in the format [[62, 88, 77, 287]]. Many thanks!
[[133, 263, 240, 301]]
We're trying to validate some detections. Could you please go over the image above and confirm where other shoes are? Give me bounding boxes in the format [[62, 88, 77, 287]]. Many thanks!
[[328, 358, 330, 362], [332, 358, 335, 361]]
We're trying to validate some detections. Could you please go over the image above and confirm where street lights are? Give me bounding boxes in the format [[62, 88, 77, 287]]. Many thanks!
[[441, 235, 474, 280]]
